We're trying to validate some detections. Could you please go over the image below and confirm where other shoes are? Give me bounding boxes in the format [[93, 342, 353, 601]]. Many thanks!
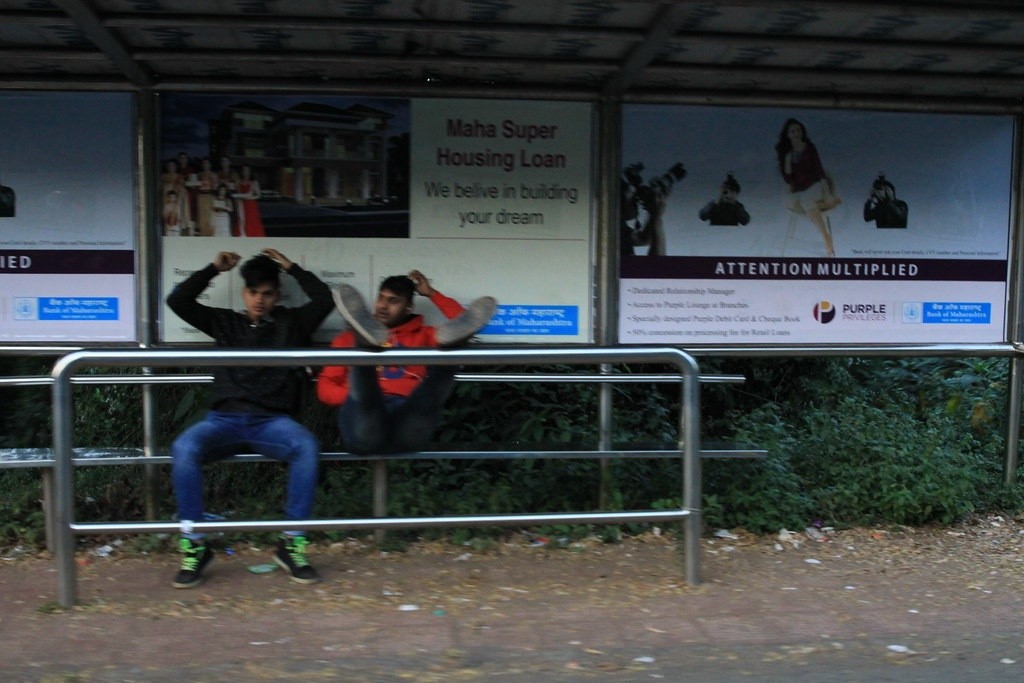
[[434, 296, 496, 350], [332, 284, 388, 347]]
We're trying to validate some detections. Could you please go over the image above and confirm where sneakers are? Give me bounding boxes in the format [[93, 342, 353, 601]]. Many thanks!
[[173, 537, 216, 588], [271, 533, 319, 583]]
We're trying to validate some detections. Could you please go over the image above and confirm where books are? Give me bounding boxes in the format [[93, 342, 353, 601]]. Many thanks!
[[241, 131, 377, 205]]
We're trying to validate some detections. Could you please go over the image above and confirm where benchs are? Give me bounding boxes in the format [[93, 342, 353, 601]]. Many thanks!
[[0, 373, 767, 552]]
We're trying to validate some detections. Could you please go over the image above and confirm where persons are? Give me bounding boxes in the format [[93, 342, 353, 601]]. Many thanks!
[[864, 181, 909, 229], [618, 177, 666, 257], [699, 177, 752, 228], [166, 247, 337, 590], [162, 150, 266, 238], [774, 117, 839, 258], [315, 270, 497, 457]]
